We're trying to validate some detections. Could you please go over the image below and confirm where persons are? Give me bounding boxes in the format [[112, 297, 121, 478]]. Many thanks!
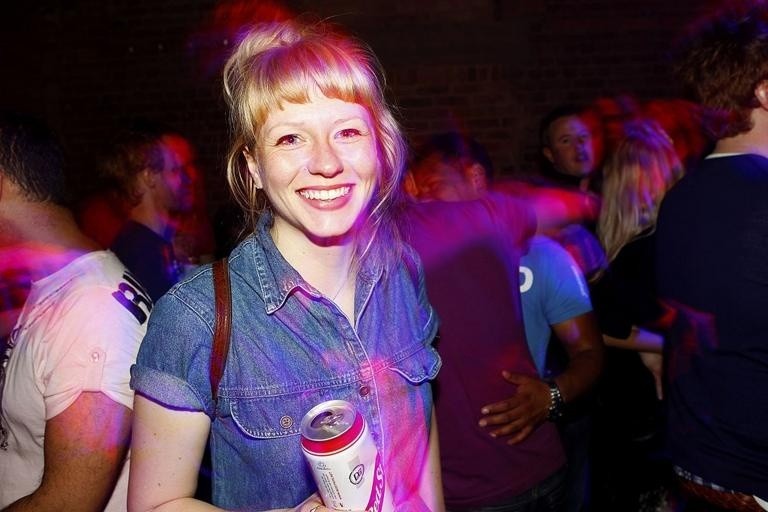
[[539, 114, 598, 190], [110, 124, 201, 306], [132, 17, 446, 512], [384, 184, 593, 511], [649, 14, 768, 511], [598, 126, 685, 511], [1, 110, 155, 511], [421, 130, 608, 511]]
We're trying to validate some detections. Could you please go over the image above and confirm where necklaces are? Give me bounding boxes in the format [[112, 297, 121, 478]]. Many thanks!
[[333, 279, 348, 301]]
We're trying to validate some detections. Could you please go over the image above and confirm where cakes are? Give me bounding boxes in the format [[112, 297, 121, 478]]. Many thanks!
[[299, 399, 397, 512]]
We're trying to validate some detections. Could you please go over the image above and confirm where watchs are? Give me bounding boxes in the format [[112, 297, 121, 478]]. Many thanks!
[[545, 380, 565, 421]]
